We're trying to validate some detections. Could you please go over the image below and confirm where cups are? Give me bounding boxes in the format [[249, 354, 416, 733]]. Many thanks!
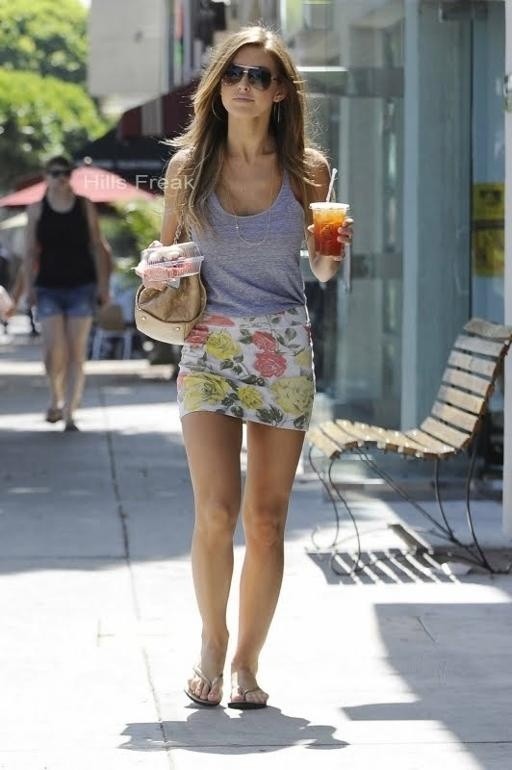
[[307, 202, 349, 257]]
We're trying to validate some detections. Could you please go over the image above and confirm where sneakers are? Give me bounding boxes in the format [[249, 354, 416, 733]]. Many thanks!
[[64, 420, 79, 431], [45, 403, 67, 423]]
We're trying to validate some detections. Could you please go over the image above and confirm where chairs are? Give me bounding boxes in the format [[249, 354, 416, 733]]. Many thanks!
[[91, 303, 140, 360]]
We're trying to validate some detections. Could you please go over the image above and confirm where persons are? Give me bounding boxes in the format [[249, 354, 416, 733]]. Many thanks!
[[133, 18, 355, 709], [22, 154, 102, 433]]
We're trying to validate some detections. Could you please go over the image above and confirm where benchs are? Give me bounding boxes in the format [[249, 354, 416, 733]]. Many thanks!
[[304, 316, 512, 576]]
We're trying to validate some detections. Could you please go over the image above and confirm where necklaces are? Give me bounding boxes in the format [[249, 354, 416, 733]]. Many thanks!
[[224, 150, 278, 246]]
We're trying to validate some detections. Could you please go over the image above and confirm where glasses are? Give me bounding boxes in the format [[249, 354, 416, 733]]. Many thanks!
[[46, 169, 71, 178], [220, 63, 281, 91]]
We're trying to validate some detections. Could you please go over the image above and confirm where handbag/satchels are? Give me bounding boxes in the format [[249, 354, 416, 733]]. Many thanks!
[[135, 274, 208, 346]]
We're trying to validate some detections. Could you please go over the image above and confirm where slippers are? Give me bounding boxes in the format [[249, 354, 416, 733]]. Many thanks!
[[183, 664, 223, 706], [228, 683, 267, 709]]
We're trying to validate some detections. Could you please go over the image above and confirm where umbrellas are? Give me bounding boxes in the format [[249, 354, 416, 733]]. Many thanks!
[[1, 165, 164, 209]]
